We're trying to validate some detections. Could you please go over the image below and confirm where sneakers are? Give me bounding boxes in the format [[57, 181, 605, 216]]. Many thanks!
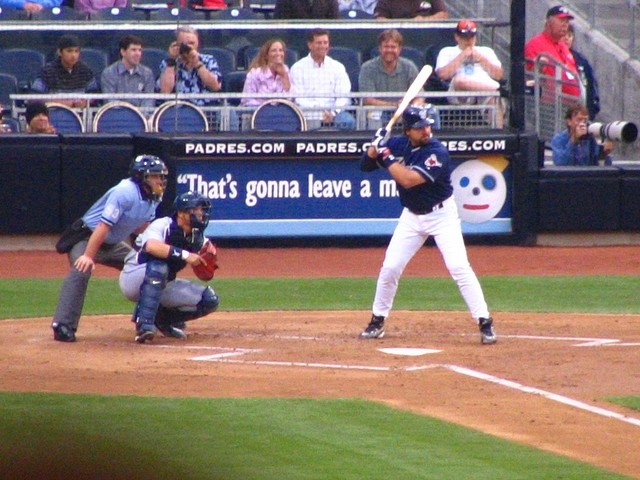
[[153, 320, 187, 341], [477, 317, 497, 344], [134, 328, 154, 344], [174, 321, 186, 329], [358, 314, 386, 339], [52, 322, 75, 342]]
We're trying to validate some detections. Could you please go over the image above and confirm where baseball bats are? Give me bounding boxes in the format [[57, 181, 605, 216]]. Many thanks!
[[385, 65, 432, 135]]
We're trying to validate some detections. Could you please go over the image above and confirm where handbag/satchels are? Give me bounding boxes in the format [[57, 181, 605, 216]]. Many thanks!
[[55, 218, 93, 254]]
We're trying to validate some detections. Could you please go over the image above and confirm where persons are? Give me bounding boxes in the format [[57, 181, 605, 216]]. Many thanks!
[[100, 34, 155, 120], [358, 29, 426, 130], [290, 28, 356, 132], [273, 0, 340, 19], [26, 102, 56, 134], [359, 104, 496, 344], [237, 38, 294, 131], [119, 190, 219, 344], [0, 0, 65, 20], [523, 5, 581, 105], [434, 19, 505, 129], [179, 0, 227, 11], [0, 104, 13, 133], [51, 154, 169, 342], [551, 104, 613, 166], [30, 34, 98, 121], [560, 23, 600, 121], [74, 0, 127, 21], [154, 27, 223, 131], [374, 0, 449, 21]]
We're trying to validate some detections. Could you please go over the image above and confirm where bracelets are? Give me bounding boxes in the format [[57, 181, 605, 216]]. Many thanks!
[[476, 55, 482, 63], [181, 250, 189, 260], [383, 155, 396, 168], [196, 63, 204, 69], [167, 245, 182, 260]]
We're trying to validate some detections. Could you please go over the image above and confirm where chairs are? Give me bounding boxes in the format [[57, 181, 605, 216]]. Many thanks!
[[51, 49, 107, 84], [0, 73, 18, 105], [93, 100, 148, 134], [244, 47, 297, 70], [26, 100, 85, 134], [370, 46, 422, 72], [2, 117, 21, 133], [426, 43, 453, 78], [222, 73, 247, 93], [139, 49, 167, 83], [326, 47, 361, 92], [148, 100, 209, 132], [0, 48, 46, 83], [251, 98, 307, 132], [199, 45, 237, 73]]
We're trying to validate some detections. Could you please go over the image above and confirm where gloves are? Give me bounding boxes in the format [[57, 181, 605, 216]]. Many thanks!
[[376, 128, 387, 138], [375, 138, 391, 156]]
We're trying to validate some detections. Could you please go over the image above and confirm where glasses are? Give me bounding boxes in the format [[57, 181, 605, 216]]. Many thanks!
[[457, 27, 477, 36]]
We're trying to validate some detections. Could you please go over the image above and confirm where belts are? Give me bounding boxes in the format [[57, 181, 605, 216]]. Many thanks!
[[408, 203, 443, 215]]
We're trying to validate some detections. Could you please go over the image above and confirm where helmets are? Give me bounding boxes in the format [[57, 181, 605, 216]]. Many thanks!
[[402, 107, 435, 128], [172, 191, 212, 233], [128, 153, 169, 203]]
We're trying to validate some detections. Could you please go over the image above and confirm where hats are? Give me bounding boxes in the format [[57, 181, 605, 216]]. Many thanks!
[[57, 33, 81, 49], [456, 20, 477, 37], [25, 102, 49, 126], [547, 6, 574, 19]]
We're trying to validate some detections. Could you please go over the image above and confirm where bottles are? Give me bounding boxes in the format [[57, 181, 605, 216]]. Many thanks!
[[465, 60, 474, 75]]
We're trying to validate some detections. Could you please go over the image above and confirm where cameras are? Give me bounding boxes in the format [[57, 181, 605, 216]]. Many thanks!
[[579, 120, 638, 144], [180, 42, 192, 54]]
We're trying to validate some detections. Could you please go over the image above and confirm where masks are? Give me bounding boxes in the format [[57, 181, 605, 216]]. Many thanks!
[[31, 119, 47, 133]]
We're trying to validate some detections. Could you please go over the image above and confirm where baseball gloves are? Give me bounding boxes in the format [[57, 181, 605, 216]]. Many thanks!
[[191, 242, 219, 282]]
